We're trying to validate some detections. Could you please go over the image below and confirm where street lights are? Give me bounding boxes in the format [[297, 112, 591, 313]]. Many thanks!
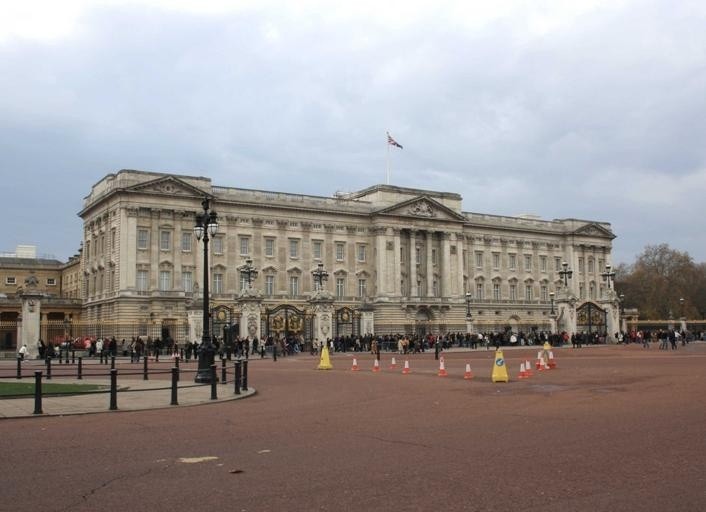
[[466, 291, 471, 316], [193, 195, 220, 383], [549, 291, 555, 314], [559, 262, 572, 293], [600, 265, 616, 296]]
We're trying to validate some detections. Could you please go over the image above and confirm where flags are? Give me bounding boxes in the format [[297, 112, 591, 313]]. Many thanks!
[[388, 134, 403, 150]]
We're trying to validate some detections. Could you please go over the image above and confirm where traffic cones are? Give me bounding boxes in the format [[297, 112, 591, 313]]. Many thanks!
[[438, 350, 449, 376], [620, 292, 625, 315], [390, 356, 398, 371], [525, 359, 534, 376], [492, 349, 508, 382], [464, 360, 474, 378], [401, 359, 411, 374], [679, 296, 684, 317], [373, 357, 381, 372], [535, 342, 557, 370], [351, 351, 360, 371], [517, 361, 527, 378], [317, 341, 333, 371]]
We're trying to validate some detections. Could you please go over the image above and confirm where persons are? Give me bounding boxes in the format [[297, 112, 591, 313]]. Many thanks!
[[18, 344, 29, 362]]
